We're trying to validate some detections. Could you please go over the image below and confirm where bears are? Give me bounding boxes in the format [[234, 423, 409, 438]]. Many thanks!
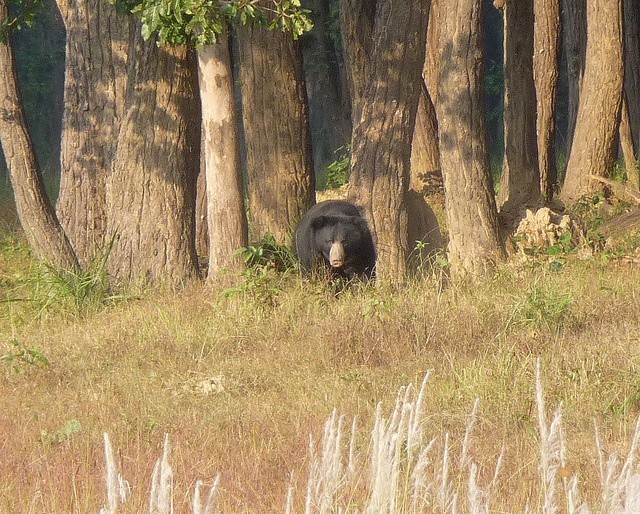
[[291, 198, 377, 300]]
